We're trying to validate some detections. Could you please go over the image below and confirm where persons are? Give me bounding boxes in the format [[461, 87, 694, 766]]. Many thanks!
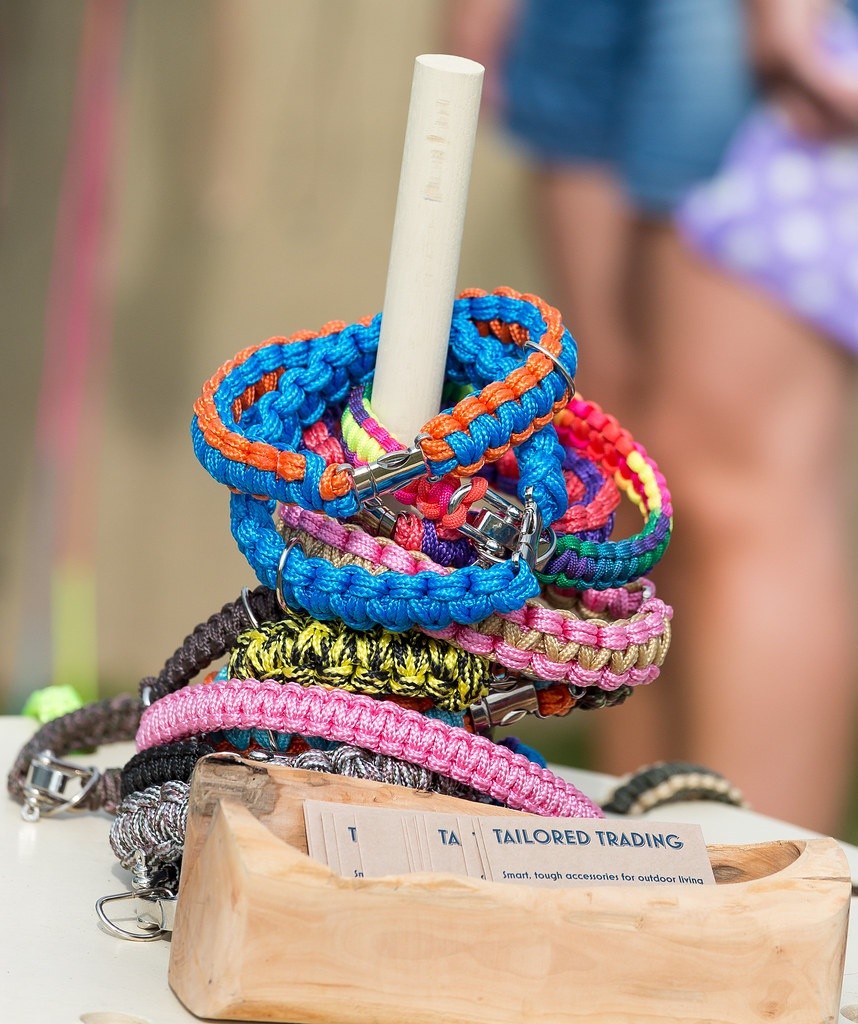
[[450, 1, 857, 853]]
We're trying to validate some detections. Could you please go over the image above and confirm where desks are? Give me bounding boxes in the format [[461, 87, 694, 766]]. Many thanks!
[[0, 716, 858, 1024]]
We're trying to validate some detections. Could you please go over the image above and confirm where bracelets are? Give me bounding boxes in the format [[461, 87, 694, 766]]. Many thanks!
[[0, 281, 748, 943]]
